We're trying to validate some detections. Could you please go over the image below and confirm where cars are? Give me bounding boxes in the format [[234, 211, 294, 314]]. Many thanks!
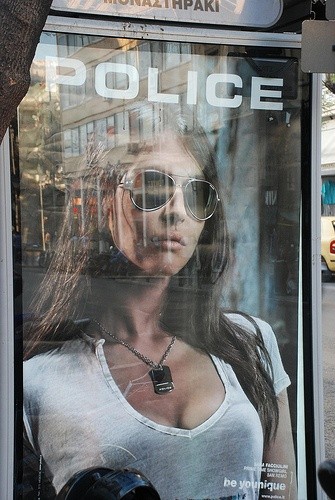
[[321, 216, 335, 283]]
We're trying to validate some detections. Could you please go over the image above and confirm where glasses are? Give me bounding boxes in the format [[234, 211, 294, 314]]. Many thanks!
[[116, 169, 221, 220]]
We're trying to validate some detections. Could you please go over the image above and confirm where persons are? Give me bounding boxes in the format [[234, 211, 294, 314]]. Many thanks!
[[19, 99, 298, 499]]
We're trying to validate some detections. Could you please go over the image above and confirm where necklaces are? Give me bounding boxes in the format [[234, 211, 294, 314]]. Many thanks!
[[90, 315, 181, 395]]
